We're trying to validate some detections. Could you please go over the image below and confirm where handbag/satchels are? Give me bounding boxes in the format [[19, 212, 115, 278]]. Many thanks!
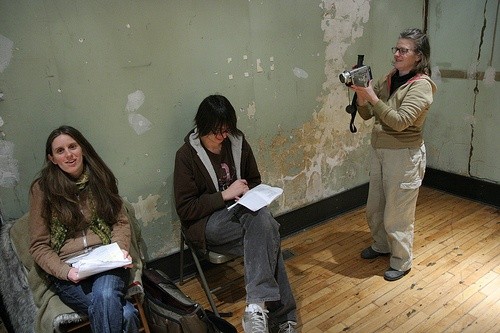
[[141, 267, 238, 333]]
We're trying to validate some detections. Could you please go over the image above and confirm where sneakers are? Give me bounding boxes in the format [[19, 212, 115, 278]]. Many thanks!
[[268, 319, 298, 333], [241, 304, 270, 333]]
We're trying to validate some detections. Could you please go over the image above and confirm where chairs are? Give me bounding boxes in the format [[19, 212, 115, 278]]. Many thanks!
[[9, 198, 152, 333], [178, 226, 244, 320]]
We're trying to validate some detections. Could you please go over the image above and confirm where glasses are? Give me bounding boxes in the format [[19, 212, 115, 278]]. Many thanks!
[[391, 47, 419, 55]]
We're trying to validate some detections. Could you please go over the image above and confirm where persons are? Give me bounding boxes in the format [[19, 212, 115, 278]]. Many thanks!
[[174, 95, 299, 333], [28, 126, 139, 333], [351, 28, 436, 281]]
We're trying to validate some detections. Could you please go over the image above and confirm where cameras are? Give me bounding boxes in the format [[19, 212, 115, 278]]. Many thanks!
[[339, 65, 373, 87]]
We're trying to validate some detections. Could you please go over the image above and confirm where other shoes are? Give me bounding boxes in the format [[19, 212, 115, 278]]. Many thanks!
[[361, 246, 390, 259], [384, 267, 411, 280]]
